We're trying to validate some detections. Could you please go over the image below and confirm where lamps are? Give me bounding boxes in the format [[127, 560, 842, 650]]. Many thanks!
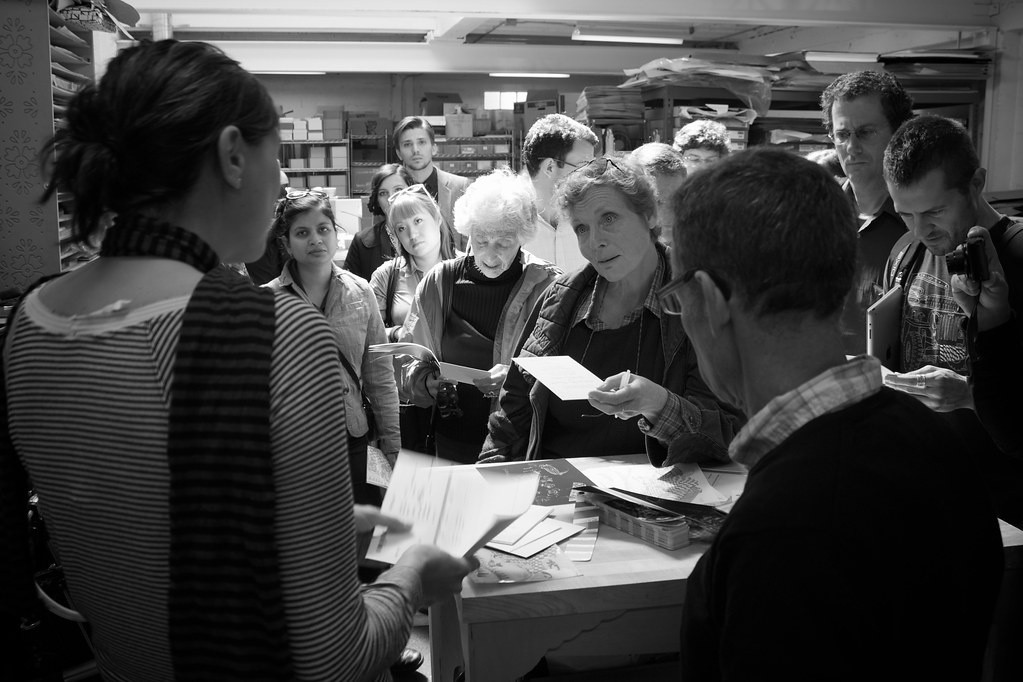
[[571, 20, 690, 44]]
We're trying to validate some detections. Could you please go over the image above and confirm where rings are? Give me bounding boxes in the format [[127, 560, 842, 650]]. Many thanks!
[[915, 374, 926, 389], [621, 405, 625, 415]]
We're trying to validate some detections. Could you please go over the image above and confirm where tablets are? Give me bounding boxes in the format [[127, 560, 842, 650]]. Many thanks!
[[866, 284, 903, 370]]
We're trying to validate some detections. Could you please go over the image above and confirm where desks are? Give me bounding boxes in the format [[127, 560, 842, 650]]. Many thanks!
[[425, 454, 1023, 682]]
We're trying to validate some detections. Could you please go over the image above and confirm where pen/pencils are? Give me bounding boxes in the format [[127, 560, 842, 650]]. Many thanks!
[[618, 370, 630, 390]]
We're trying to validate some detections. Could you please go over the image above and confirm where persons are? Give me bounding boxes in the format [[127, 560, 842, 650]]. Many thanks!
[[0, 37, 481, 682], [820, 69, 921, 362], [475, 151, 751, 468], [393, 162, 568, 461], [881, 114, 1023, 470], [254, 186, 405, 503], [245, 113, 850, 463], [656, 148, 1023, 682]]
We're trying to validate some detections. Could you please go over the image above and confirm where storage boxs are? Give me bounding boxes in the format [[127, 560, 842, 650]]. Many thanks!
[[473, 119, 491, 133], [513, 89, 558, 140], [442, 101, 473, 138], [432, 143, 511, 171], [277, 104, 390, 252], [418, 93, 463, 116]]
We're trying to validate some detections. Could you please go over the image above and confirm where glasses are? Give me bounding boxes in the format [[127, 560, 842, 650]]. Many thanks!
[[558, 158, 626, 189], [828, 123, 892, 145], [682, 156, 718, 168], [657, 265, 732, 315], [387, 184, 436, 207], [281, 190, 329, 217], [543, 157, 588, 168]]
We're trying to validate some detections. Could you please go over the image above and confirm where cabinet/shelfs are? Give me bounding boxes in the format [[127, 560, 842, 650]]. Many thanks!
[[403, 127, 515, 180], [0, 0, 117, 293], [587, 80, 986, 159], [350, 129, 388, 197], [279, 129, 351, 199]]
